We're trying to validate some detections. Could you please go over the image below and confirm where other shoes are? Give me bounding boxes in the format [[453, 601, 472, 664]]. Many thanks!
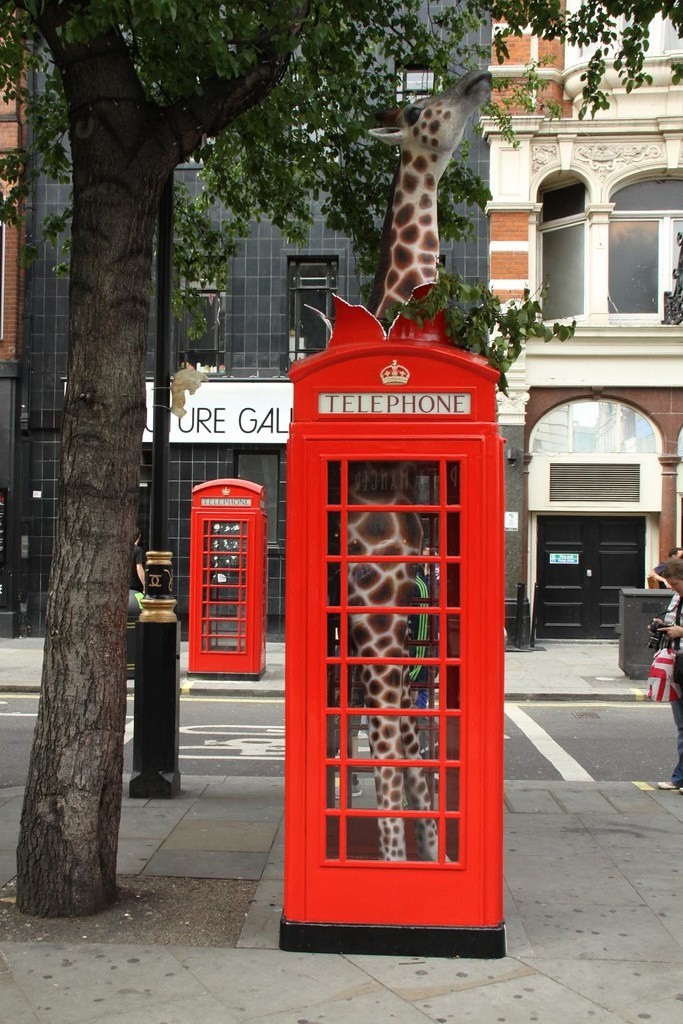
[[334, 781, 362, 799], [657, 781, 683, 793]]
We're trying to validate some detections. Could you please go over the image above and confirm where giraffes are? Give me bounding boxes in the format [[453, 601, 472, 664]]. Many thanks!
[[334, 64, 496, 865]]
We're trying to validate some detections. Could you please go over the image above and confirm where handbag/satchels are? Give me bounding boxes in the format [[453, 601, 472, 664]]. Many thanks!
[[647, 648, 681, 703]]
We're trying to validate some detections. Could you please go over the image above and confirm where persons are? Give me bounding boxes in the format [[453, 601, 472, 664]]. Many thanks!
[[651, 547, 683, 589], [334, 547, 441, 799], [647, 560, 683, 794], [129, 527, 146, 595]]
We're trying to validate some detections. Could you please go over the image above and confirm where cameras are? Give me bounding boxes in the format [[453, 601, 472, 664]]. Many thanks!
[[646, 620, 672, 652]]
